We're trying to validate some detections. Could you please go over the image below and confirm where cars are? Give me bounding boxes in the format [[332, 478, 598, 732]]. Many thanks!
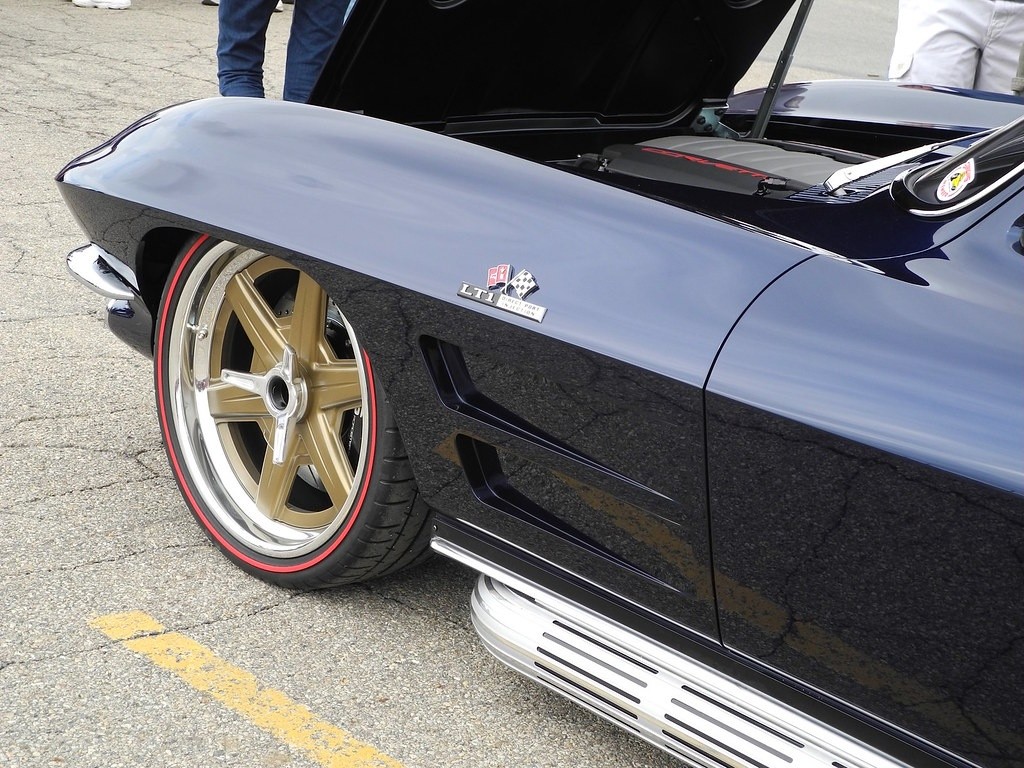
[[54, 1, 1024, 768]]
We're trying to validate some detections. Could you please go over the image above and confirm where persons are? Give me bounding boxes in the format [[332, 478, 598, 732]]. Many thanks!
[[889, 0, 1024, 97], [72, 0, 131, 10], [216, 0, 353, 102]]
